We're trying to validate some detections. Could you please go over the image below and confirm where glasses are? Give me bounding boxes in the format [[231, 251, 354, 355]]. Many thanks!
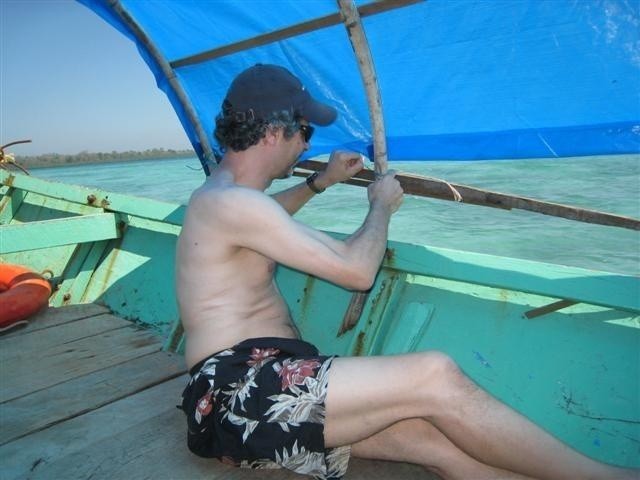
[[300, 123, 314, 142]]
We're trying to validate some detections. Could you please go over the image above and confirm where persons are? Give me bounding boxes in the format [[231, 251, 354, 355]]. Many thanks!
[[174, 64, 639, 480]]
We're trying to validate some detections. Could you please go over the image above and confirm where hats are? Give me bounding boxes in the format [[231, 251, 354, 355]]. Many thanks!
[[224, 63, 338, 127]]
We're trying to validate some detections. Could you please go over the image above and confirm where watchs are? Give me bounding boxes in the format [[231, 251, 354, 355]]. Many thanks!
[[306, 172, 325, 194]]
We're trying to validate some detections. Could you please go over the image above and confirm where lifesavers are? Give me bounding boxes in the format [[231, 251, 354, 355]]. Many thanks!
[[0, 262, 53, 328]]
